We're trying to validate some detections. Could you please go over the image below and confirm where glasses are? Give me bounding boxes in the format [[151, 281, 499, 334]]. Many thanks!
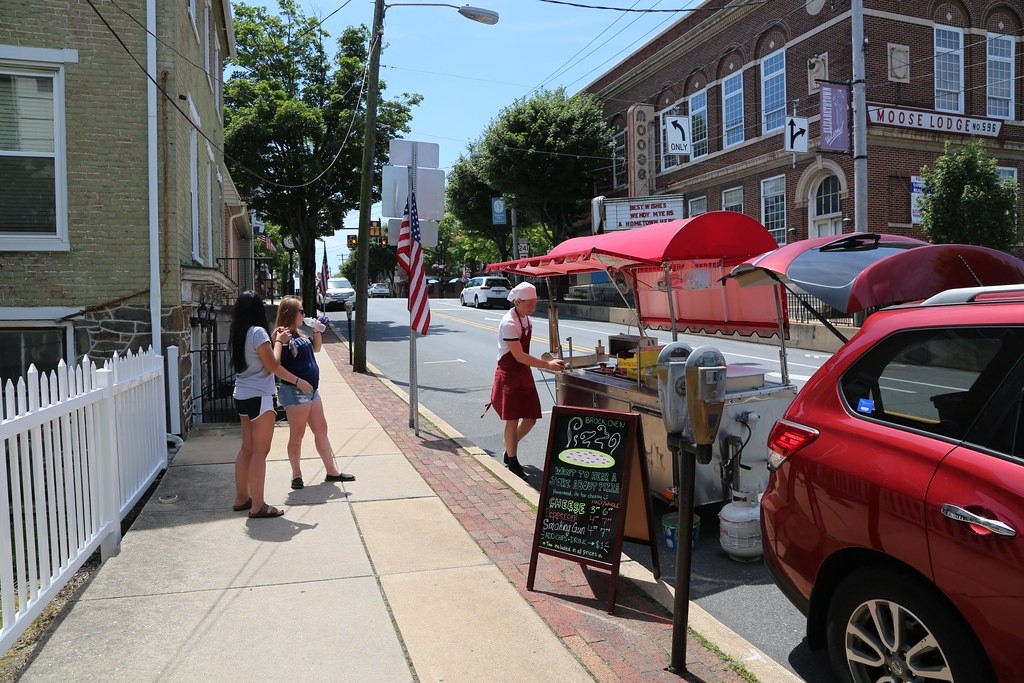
[[297, 309, 304, 315]]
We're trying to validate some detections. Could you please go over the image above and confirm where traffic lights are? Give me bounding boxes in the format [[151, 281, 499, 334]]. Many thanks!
[[382, 237, 386, 248], [443, 273, 446, 277], [451, 233, 455, 244], [349, 236, 356, 248]]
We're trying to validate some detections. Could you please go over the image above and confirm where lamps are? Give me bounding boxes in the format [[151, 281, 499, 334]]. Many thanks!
[[190, 297, 216, 328], [808, 53, 817, 70], [843, 213, 852, 226], [787, 224, 796, 235]]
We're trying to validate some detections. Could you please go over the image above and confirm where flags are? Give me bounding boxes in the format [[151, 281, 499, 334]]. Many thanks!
[[397, 177, 431, 336], [319, 245, 330, 297]]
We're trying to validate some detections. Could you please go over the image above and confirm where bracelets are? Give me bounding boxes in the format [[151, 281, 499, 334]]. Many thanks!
[[275, 340, 284, 345], [313, 330, 320, 333], [295, 377, 299, 387]]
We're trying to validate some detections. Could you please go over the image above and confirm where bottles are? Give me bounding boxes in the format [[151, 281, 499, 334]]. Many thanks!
[[645, 362, 654, 388], [303, 318, 326, 332]]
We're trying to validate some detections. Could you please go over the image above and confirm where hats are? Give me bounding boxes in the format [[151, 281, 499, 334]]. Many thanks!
[[507, 282, 537, 302]]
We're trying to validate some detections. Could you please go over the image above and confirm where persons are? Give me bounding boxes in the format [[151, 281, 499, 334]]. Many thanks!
[[491, 281, 566, 480], [227, 291, 292, 518], [276, 297, 355, 489]]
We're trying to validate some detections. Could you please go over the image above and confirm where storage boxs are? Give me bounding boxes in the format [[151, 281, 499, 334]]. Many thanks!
[[617, 345, 666, 380]]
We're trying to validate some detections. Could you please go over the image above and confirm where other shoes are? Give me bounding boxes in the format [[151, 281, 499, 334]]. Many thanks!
[[290, 477, 304, 489], [504, 451, 527, 479], [325, 473, 356, 482]]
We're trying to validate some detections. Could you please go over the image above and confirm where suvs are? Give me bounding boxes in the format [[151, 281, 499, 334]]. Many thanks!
[[717, 231, 1024, 683], [317, 277, 356, 311], [368, 283, 390, 298], [460, 276, 513, 308]]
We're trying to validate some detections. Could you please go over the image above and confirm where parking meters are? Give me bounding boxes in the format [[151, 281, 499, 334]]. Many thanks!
[[344, 301, 354, 364], [655, 339, 729, 672]]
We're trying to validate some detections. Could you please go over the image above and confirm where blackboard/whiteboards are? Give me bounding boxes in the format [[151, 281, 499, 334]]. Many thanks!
[[533, 406, 669, 572]]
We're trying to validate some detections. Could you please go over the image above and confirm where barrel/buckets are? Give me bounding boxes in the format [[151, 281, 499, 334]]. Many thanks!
[[661, 512, 700, 554]]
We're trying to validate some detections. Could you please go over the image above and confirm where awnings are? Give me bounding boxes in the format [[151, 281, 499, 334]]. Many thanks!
[[261, 230, 277, 252]]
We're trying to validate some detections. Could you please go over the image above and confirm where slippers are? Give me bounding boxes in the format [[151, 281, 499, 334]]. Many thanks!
[[233, 497, 252, 512], [248, 503, 284, 518]]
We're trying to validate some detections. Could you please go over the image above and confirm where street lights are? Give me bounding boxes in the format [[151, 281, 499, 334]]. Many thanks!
[[355, 3, 499, 371]]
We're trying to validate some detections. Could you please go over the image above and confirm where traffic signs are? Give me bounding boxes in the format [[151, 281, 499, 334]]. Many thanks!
[[664, 115, 692, 155]]
